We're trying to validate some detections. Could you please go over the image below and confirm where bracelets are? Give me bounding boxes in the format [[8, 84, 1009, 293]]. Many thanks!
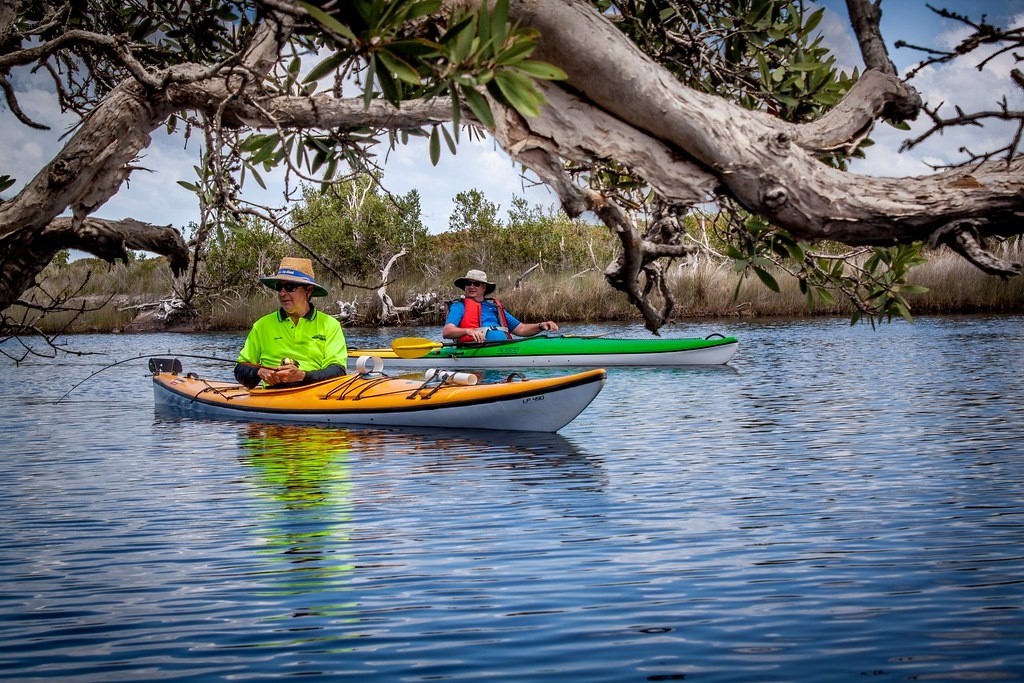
[[538, 323, 545, 332]]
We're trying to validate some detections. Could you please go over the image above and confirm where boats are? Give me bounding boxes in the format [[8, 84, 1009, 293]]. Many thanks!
[[152, 370, 609, 435], [347, 333, 741, 366]]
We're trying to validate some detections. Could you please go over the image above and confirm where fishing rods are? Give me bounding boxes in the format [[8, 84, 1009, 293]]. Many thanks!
[[49, 348, 282, 408]]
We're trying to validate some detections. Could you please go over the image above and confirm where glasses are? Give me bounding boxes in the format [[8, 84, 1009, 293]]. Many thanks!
[[275, 280, 307, 292], [464, 281, 485, 287]]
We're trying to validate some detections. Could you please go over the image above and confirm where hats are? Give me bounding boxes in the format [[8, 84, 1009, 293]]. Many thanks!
[[259, 257, 328, 297], [454, 269, 496, 296]]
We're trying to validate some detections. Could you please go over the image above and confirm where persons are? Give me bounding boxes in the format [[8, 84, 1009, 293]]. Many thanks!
[[234, 256, 349, 390], [443, 270, 558, 348]]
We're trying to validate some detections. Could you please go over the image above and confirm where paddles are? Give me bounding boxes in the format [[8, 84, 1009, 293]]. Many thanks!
[[389, 328, 612, 362]]
